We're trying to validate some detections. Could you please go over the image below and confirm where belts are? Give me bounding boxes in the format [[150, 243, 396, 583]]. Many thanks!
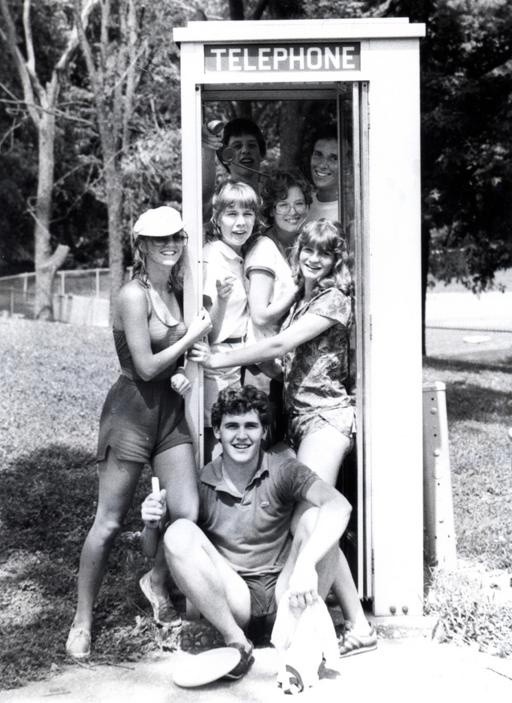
[[224, 335, 247, 344]]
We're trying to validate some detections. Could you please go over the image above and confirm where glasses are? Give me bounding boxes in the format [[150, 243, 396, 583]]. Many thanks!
[[274, 197, 309, 213], [151, 229, 189, 248]]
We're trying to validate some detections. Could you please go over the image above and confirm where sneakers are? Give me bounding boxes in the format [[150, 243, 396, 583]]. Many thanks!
[[67, 621, 91, 659], [137, 572, 183, 629], [223, 639, 255, 681], [275, 662, 304, 696], [332, 623, 377, 657]]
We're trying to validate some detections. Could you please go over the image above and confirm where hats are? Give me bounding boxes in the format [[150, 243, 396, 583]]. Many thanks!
[[134, 205, 183, 237]]
